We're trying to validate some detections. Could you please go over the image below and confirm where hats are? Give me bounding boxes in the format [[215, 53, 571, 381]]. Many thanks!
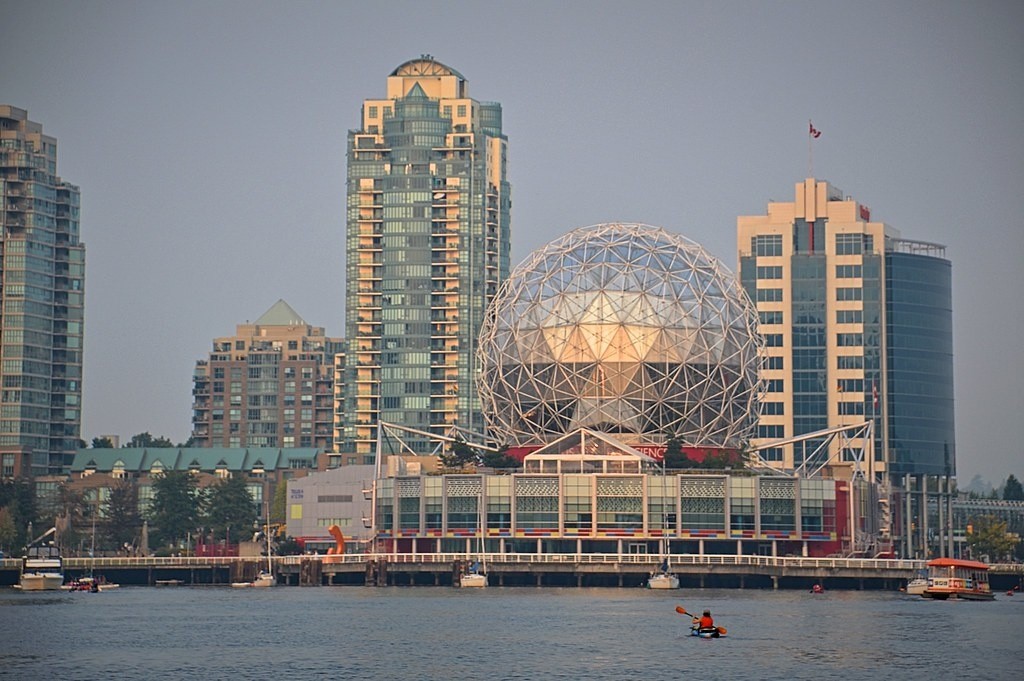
[[704, 609, 710, 614]]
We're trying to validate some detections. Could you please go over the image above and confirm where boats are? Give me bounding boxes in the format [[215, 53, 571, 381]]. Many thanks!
[[907, 578, 930, 598], [231, 582, 251, 589], [13, 583, 120, 589], [20, 572, 65, 590], [690, 624, 717, 638], [925, 558, 997, 602]]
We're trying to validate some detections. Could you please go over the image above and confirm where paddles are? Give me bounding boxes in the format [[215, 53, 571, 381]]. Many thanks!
[[1001, 585, 1019, 595], [676, 606, 727, 634]]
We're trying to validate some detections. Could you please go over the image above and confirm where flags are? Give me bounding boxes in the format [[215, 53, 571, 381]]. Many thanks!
[[810, 124, 821, 138]]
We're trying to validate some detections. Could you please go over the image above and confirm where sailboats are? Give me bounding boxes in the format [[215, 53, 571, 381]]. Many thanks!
[[252, 502, 278, 589], [646, 461, 684, 590], [459, 494, 489, 588]]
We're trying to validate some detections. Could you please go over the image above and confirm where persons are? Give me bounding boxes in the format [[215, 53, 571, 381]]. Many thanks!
[[692, 609, 713, 628]]
